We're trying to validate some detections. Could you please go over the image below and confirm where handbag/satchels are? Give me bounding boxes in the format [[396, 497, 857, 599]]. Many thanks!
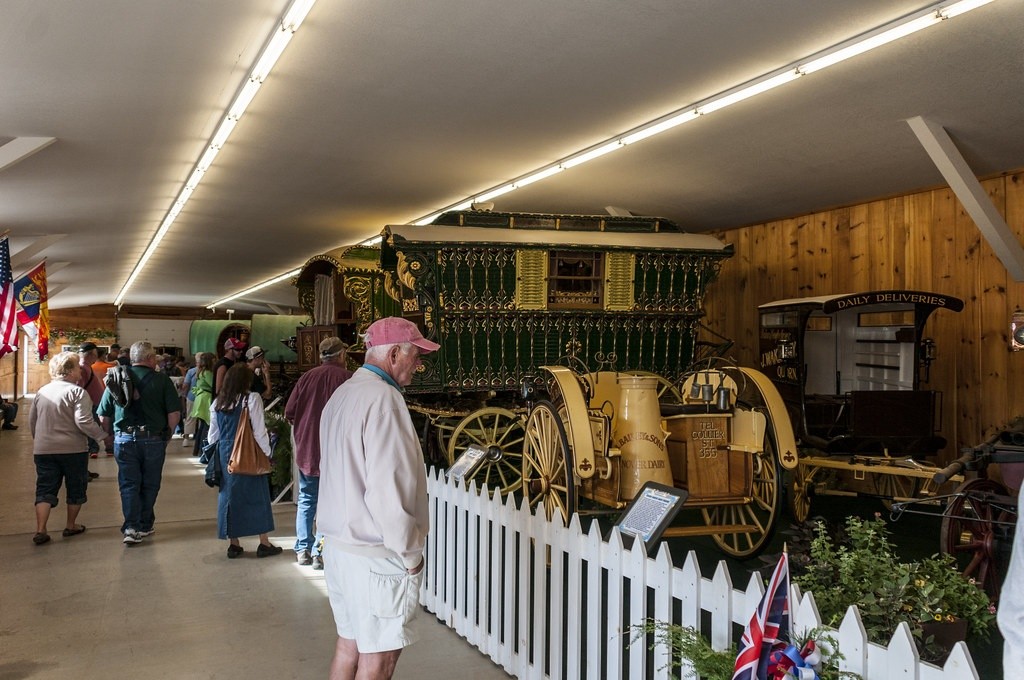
[[227, 393, 272, 475]]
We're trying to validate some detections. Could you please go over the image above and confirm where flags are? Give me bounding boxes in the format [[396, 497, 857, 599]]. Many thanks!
[[730, 541, 796, 680], [0, 228, 20, 361], [12, 256, 49, 364]]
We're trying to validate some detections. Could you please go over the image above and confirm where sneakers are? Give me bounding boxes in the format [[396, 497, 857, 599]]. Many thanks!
[[123, 529, 143, 542], [138, 526, 155, 536]]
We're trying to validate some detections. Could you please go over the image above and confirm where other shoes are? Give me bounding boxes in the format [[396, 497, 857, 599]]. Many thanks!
[[193, 450, 202, 457], [172, 434, 183, 439], [2, 424, 18, 430], [90, 452, 97, 458], [227, 544, 243, 558], [257, 543, 283, 558], [107, 452, 114, 455], [312, 556, 323, 570], [182, 439, 189, 446], [296, 550, 311, 565], [63, 524, 86, 537], [33, 533, 50, 543], [87, 471, 99, 481]]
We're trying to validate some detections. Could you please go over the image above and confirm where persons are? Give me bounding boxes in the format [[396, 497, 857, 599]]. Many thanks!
[[284, 336, 355, 570], [0, 394, 19, 430], [207, 361, 283, 559], [95, 341, 181, 546], [315, 316, 441, 680], [28, 351, 115, 545], [78, 339, 273, 484]]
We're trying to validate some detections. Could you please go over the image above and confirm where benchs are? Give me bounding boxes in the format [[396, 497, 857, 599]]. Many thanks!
[[805, 393, 850, 432]]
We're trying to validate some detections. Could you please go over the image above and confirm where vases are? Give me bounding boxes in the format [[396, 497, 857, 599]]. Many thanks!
[[918, 618, 971, 665]]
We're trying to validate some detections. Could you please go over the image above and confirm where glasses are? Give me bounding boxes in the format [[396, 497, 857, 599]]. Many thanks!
[[230, 348, 241, 351]]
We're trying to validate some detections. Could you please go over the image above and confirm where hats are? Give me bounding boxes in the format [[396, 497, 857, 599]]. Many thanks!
[[79, 342, 99, 352], [366, 316, 441, 354], [246, 346, 265, 359], [111, 344, 121, 348], [319, 337, 349, 356], [195, 352, 204, 362], [224, 338, 246, 349]]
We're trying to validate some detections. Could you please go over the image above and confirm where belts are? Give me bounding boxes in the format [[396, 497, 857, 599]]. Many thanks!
[[117, 431, 160, 436]]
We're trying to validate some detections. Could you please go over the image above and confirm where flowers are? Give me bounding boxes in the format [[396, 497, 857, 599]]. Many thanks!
[[911, 552, 997, 646]]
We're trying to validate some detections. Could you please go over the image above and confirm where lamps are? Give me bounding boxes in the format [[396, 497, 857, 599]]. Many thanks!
[[112, 1, 995, 309]]
